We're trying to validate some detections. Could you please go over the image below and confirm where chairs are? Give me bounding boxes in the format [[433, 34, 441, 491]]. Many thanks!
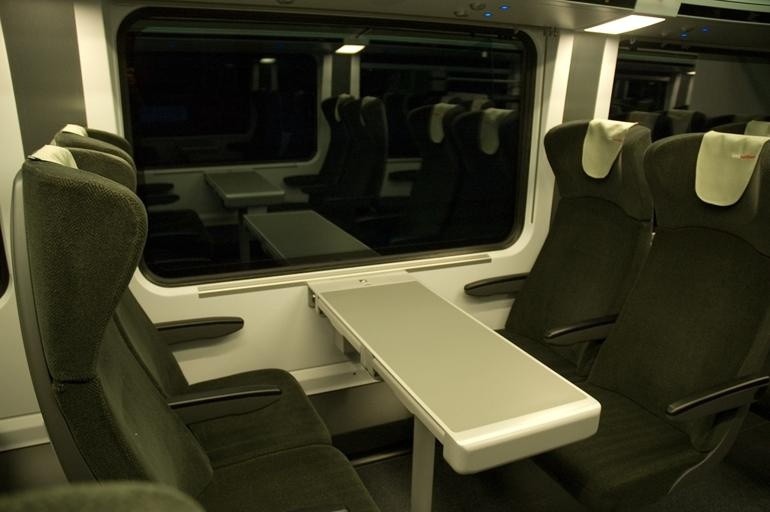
[[610, 105, 737, 145]]
